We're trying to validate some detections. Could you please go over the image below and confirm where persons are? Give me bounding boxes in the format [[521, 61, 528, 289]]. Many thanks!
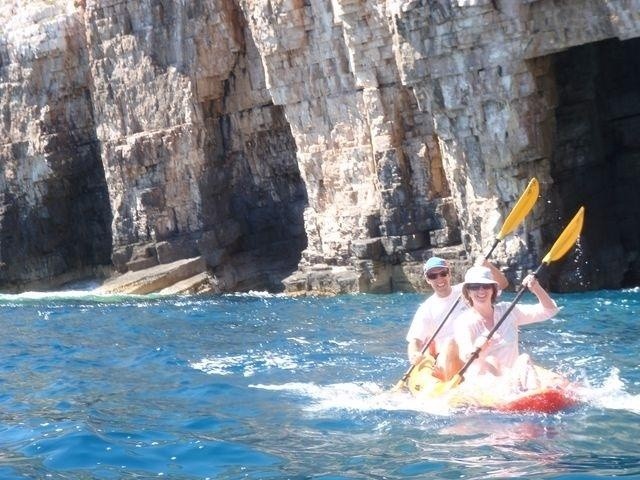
[[436, 413, 556, 443], [404, 254, 510, 366], [440, 265, 560, 402]]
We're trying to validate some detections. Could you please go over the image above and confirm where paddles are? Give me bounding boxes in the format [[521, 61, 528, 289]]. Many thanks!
[[428, 206, 585, 402], [384, 177, 540, 398]]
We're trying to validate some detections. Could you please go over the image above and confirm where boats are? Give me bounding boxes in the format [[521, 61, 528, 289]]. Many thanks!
[[405, 357, 569, 413]]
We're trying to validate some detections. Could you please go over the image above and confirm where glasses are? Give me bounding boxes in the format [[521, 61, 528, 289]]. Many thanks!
[[426, 268, 450, 280], [466, 285, 495, 291]]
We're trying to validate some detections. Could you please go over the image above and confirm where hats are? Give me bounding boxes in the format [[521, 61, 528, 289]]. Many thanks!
[[460, 265, 503, 300], [422, 256, 449, 275]]
